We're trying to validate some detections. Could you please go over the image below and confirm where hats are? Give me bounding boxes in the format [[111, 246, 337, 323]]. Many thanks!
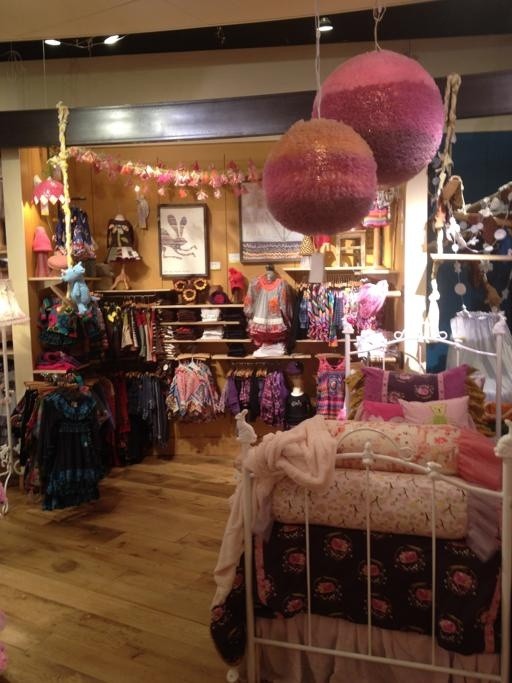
[[286, 361, 303, 374], [228, 268, 249, 293]]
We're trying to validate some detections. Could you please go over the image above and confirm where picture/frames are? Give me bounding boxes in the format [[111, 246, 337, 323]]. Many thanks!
[[238, 178, 311, 264], [156, 202, 210, 278]]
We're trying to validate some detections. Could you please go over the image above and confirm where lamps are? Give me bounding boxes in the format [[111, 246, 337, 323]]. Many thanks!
[[134, 185, 149, 229], [0, 278, 30, 516], [317, 16, 334, 32], [44, 38, 61, 46], [29, 144, 65, 216], [99, 34, 121, 44]]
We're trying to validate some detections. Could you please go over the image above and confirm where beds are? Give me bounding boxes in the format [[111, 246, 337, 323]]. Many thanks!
[[211, 315, 512, 683]]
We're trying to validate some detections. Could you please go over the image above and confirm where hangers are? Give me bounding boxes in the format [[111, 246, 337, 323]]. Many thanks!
[[23, 373, 92, 398], [225, 361, 273, 377], [96, 293, 158, 305], [125, 362, 173, 377], [315, 347, 353, 361], [328, 269, 362, 288], [175, 356, 210, 366], [295, 276, 329, 289], [59, 196, 88, 211], [255, 262, 287, 282]]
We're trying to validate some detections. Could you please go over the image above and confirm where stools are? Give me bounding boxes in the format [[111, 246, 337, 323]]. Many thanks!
[[334, 229, 366, 268]]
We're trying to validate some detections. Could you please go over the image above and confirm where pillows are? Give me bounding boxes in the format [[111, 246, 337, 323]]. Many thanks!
[[360, 363, 478, 402], [360, 400, 404, 421], [397, 395, 469, 429]]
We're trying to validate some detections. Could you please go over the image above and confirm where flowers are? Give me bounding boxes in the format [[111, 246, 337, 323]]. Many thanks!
[[174, 278, 207, 305]]
